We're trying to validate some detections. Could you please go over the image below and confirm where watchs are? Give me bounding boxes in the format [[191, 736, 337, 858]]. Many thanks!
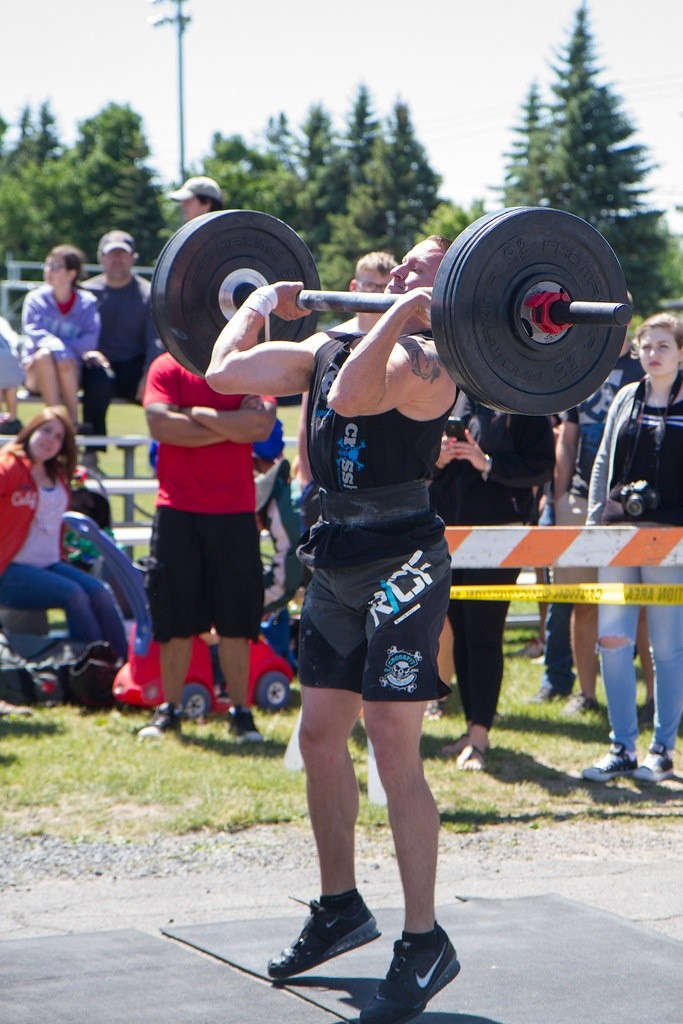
[[485, 454, 490, 460]]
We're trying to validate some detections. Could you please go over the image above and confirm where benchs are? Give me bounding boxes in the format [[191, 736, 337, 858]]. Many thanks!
[[0, 389, 160, 539]]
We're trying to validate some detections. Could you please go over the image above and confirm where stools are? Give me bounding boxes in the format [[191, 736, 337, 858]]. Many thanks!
[[0, 607, 49, 637]]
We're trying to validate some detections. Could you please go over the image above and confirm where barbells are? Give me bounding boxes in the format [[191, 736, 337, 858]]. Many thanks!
[[149, 207, 635, 418]]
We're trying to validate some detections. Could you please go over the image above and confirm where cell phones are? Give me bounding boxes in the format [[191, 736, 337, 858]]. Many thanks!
[[445, 417, 467, 443]]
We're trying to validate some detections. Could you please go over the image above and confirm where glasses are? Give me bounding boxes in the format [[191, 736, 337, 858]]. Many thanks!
[[358, 281, 388, 292]]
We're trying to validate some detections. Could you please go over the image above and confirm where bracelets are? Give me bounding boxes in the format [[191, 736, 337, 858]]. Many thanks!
[[242, 284, 278, 317]]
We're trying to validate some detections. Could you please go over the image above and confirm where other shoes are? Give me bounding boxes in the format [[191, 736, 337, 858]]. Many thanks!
[[527, 687, 571, 704], [424, 700, 446, 721], [561, 693, 599, 716]]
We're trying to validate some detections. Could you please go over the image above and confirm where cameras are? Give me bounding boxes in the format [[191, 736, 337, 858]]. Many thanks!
[[621, 480, 658, 516]]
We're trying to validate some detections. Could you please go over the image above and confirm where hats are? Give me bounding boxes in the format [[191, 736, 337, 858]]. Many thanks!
[[98, 230, 135, 255], [170, 176, 223, 201]]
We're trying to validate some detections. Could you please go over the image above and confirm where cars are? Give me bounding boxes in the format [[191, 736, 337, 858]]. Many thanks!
[[111, 608, 298, 716]]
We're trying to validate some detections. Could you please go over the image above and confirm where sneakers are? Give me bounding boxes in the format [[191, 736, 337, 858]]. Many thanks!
[[137, 702, 184, 736], [227, 705, 263, 745], [582, 742, 638, 782], [267, 896, 382, 978], [633, 744, 673, 782], [360, 920, 460, 1024]]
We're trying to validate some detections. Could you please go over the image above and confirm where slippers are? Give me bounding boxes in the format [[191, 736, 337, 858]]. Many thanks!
[[457, 743, 490, 771], [442, 734, 471, 756]]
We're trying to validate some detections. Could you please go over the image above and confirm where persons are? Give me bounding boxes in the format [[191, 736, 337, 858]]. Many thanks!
[[205, 232, 460, 1024], [349, 249, 556, 771], [137, 353, 277, 743], [520, 313, 683, 781], [168, 175, 223, 219], [1, 404, 129, 661], [0, 229, 154, 452]]
